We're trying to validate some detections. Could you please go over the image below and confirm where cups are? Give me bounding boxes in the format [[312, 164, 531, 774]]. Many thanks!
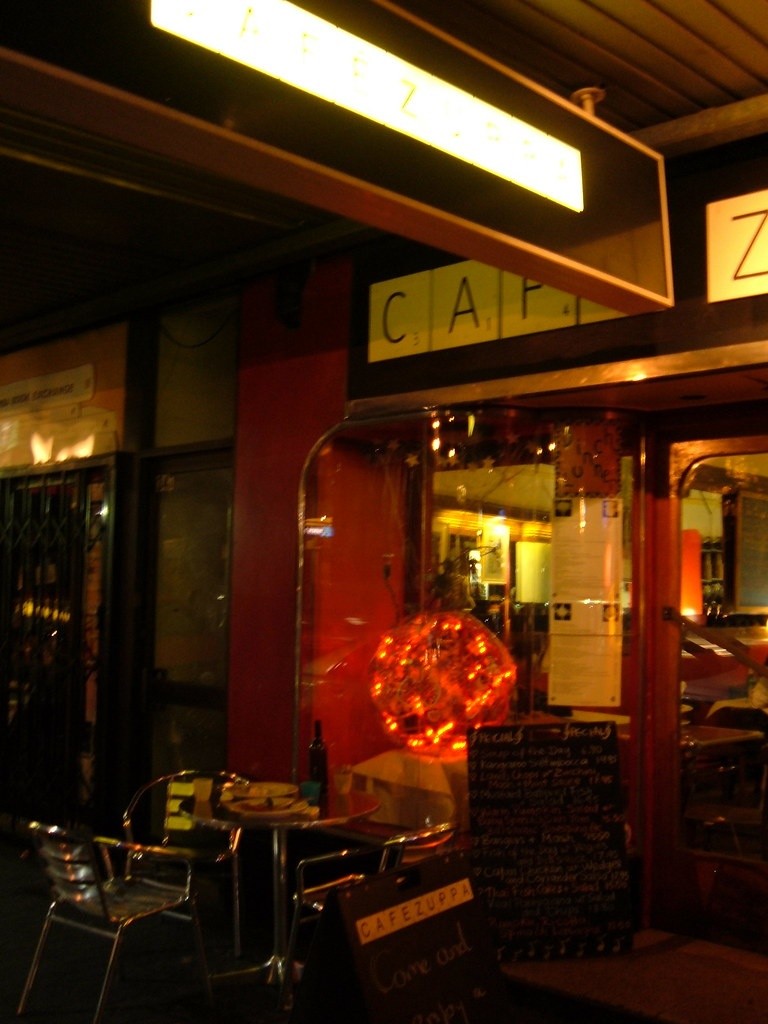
[[194, 778, 212, 801], [301, 782, 321, 806], [333, 772, 353, 795]]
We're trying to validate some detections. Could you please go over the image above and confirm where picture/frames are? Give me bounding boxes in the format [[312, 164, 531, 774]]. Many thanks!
[[480, 524, 510, 584]]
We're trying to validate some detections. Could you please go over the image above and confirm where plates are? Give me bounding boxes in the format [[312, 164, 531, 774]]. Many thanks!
[[229, 798, 308, 818], [231, 782, 298, 799]]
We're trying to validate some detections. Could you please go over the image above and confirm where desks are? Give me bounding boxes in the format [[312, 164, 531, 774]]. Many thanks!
[[706, 698, 768, 720], [503, 708, 629, 725], [616, 723, 764, 807], [179, 778, 382, 978]]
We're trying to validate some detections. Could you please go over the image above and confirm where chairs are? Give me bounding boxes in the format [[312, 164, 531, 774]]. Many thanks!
[[18, 821, 215, 1024], [123, 770, 250, 957], [707, 706, 768, 805], [278, 819, 461, 1024], [685, 764, 768, 856]]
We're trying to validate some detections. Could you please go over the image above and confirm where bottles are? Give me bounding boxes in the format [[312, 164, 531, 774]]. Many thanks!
[[307, 720, 329, 799]]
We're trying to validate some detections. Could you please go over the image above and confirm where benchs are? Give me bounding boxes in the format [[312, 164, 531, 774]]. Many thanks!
[[322, 823, 468, 851], [506, 927, 768, 1024]]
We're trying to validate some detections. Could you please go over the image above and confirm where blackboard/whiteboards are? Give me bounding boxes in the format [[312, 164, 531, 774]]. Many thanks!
[[334, 853, 523, 1024], [465, 720, 632, 962]]
